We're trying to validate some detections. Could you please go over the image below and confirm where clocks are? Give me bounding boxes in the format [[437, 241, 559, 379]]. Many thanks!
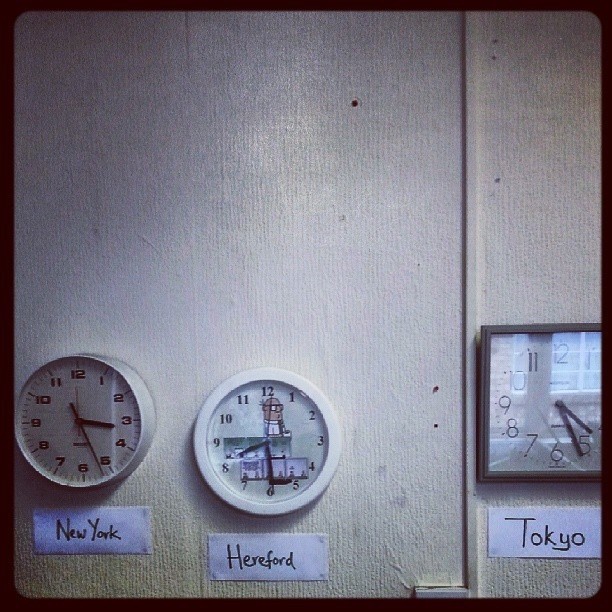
[[478, 322, 604, 482], [12, 354, 158, 490], [193, 366, 343, 518]]
